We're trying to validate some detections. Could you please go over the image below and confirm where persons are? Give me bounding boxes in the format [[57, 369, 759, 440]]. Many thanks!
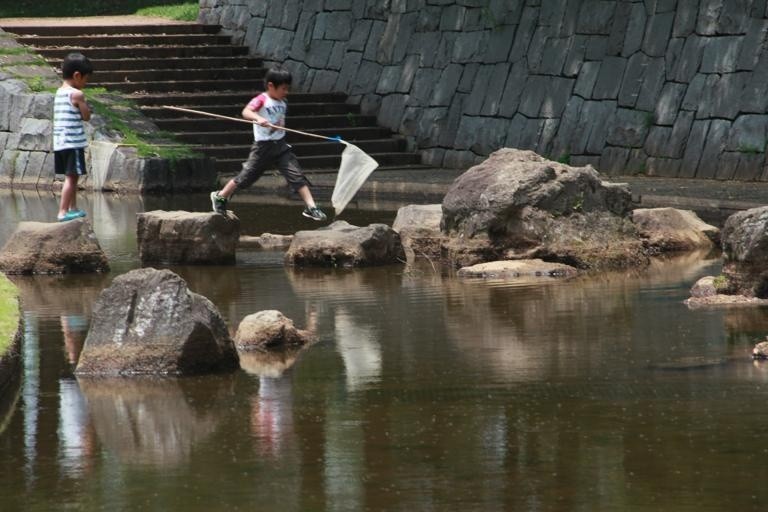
[[53, 54, 91, 220], [210, 63, 327, 223]]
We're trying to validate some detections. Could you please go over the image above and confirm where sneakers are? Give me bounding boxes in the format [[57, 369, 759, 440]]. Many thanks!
[[300, 205, 328, 222], [209, 190, 228, 217]]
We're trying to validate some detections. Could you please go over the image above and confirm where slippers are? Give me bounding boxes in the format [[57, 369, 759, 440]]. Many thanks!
[[57, 207, 87, 222]]
